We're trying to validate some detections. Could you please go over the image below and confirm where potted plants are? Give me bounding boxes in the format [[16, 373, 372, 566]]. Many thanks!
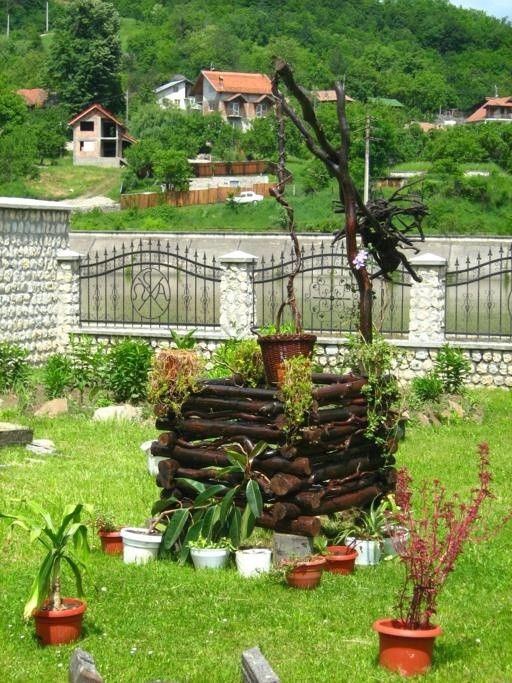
[[185, 535, 237, 572], [234, 528, 275, 578], [334, 495, 413, 566], [313, 534, 357, 577], [379, 515, 410, 554], [369, 440, 512, 677], [94, 515, 124, 555], [279, 552, 325, 588], [120, 440, 267, 569], [0, 495, 93, 645]]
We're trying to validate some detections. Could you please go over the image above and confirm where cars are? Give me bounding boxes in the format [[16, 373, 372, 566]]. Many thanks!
[[225, 191, 265, 207]]
